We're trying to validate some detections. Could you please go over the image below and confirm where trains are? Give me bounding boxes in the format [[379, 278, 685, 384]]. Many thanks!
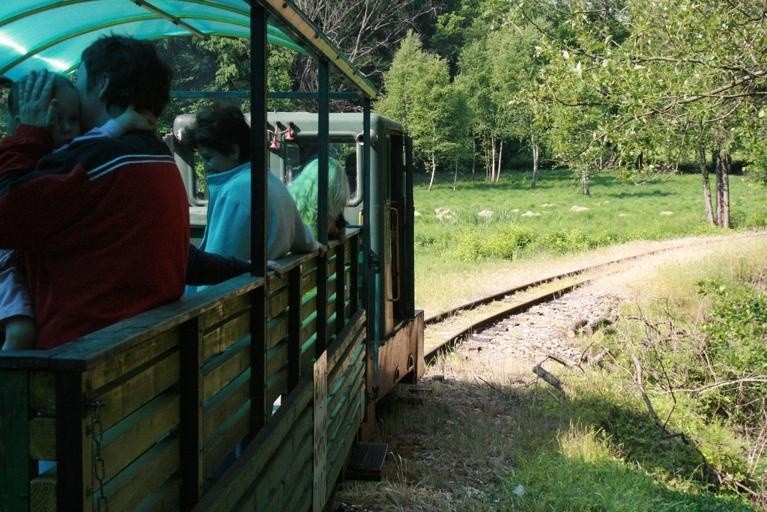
[[0, 0, 426, 512]]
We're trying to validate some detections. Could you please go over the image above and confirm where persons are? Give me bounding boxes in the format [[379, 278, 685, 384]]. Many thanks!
[[186, 241, 284, 286], [181, 103, 327, 301], [0, 31, 190, 350], [2, 73, 155, 351], [289, 156, 350, 242]]
